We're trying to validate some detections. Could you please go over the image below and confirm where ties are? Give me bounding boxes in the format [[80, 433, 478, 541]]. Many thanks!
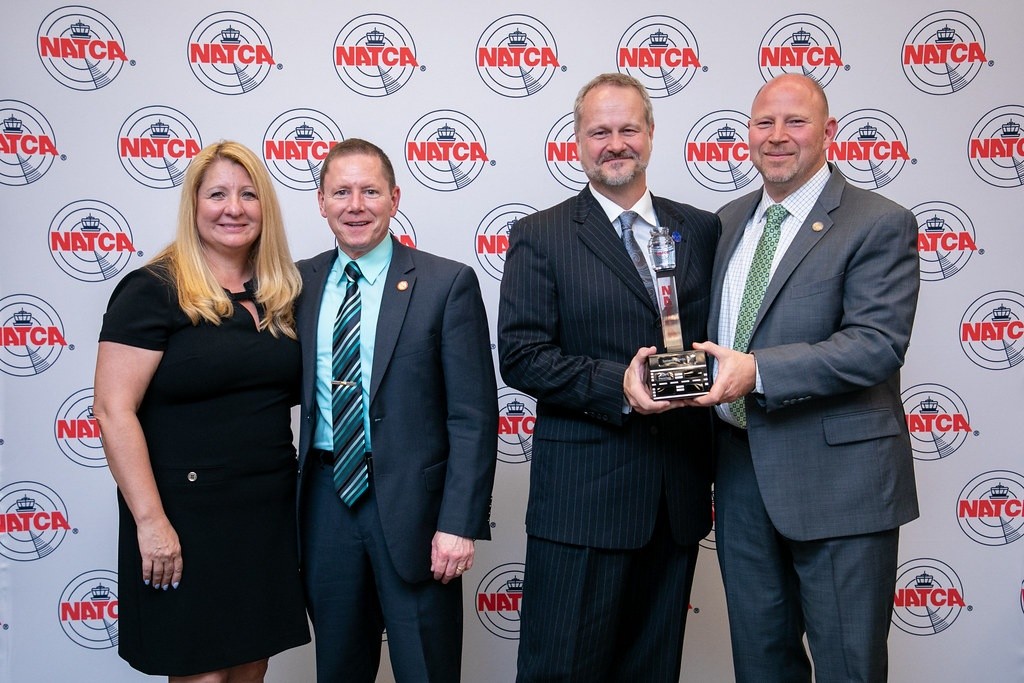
[[615, 210, 661, 321], [332, 262, 369, 508], [728, 204, 792, 430]]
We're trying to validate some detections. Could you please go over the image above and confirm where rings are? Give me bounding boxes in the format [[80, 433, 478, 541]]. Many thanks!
[[456, 567, 466, 574], [716, 402, 721, 406]]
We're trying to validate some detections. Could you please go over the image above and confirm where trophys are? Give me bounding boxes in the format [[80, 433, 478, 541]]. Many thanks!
[[642, 226, 713, 401]]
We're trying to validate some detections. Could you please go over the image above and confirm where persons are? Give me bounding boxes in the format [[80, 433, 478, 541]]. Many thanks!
[[692, 73, 920, 683], [496, 73, 723, 683], [93, 140, 312, 683], [289, 138, 499, 683]]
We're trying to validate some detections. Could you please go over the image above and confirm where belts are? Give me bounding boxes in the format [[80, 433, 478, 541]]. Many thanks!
[[311, 449, 373, 475]]
